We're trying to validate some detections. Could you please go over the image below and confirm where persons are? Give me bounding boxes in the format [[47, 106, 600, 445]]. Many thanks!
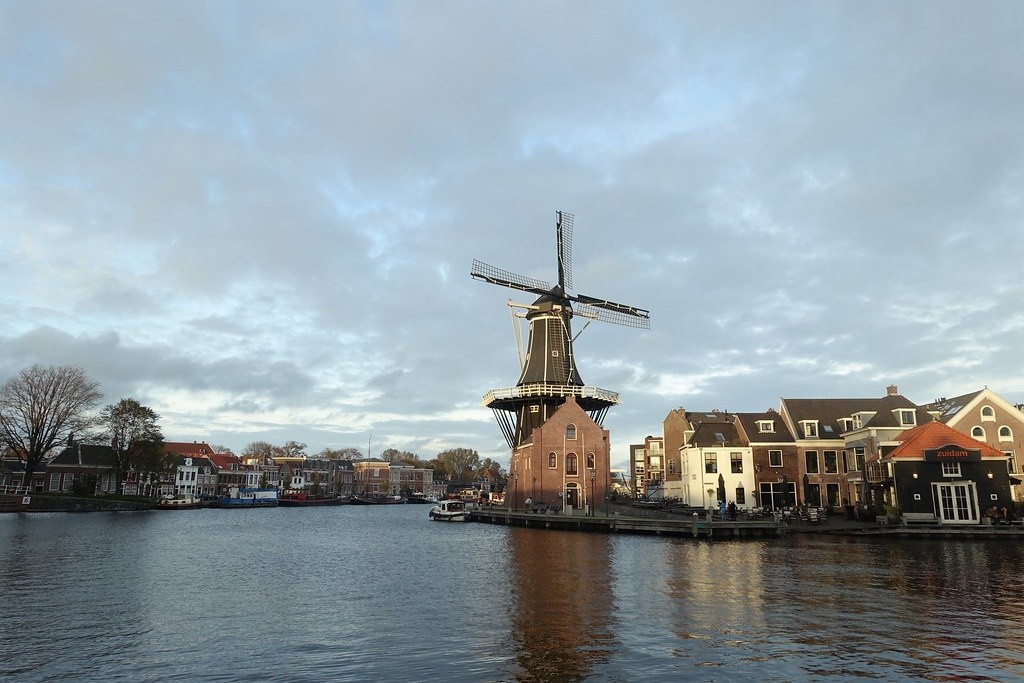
[[524, 496, 532, 514], [718, 500, 738, 521], [984, 506, 1017, 527]]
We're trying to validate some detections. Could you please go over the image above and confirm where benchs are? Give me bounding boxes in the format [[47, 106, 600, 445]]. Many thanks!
[[900, 512, 942, 526], [984, 514, 1024, 524]]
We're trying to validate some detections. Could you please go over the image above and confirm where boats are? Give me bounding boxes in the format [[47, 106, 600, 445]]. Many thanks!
[[279, 494, 342, 506], [428, 499, 472, 524], [217, 488, 280, 508], [159, 495, 203, 511]]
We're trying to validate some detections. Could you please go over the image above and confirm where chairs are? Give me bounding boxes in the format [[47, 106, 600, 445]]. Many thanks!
[[784, 511, 791, 522], [876, 516, 885, 524], [753, 508, 762, 519], [799, 511, 809, 526], [808, 508, 824, 512], [773, 512, 787, 526], [810, 512, 819, 525], [746, 510, 754, 520], [533, 504, 548, 514], [736, 507, 743, 517], [758, 507, 767, 517], [799, 507, 807, 517], [820, 510, 828, 524]]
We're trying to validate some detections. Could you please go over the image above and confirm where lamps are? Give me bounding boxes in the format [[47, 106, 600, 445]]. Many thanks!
[[988, 468, 993, 478], [912, 468, 918, 479]]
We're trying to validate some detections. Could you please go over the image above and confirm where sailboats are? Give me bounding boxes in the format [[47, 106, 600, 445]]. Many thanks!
[[348, 436, 408, 504]]
